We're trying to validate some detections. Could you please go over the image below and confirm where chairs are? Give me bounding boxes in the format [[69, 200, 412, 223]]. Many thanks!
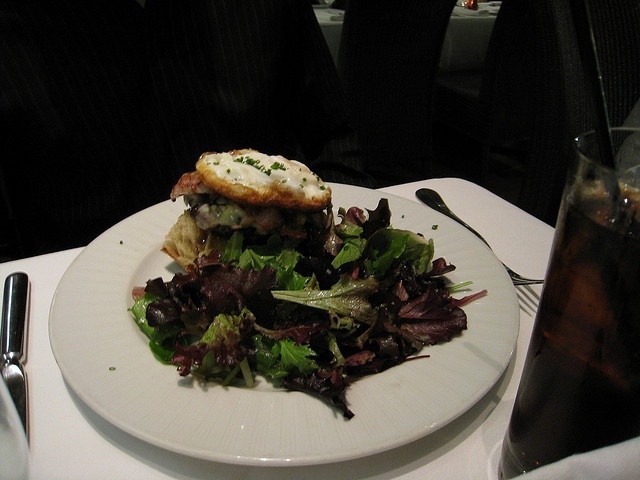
[[517, 1, 639, 224], [336, 1, 456, 180], [429, 1, 537, 193]]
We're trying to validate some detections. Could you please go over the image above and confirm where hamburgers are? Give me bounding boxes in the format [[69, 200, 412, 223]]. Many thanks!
[[165, 146, 332, 271]]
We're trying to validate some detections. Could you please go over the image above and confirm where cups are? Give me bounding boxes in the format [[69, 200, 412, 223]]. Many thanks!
[[496, 126, 640, 479]]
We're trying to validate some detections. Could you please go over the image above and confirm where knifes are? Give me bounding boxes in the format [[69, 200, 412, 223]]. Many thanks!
[[0, 272, 31, 437]]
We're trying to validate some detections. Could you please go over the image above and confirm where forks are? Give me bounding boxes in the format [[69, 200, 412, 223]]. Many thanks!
[[415, 188, 545, 286]]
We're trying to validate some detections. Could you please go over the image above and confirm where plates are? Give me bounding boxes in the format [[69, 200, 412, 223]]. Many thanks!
[[48, 181, 520, 467]]
